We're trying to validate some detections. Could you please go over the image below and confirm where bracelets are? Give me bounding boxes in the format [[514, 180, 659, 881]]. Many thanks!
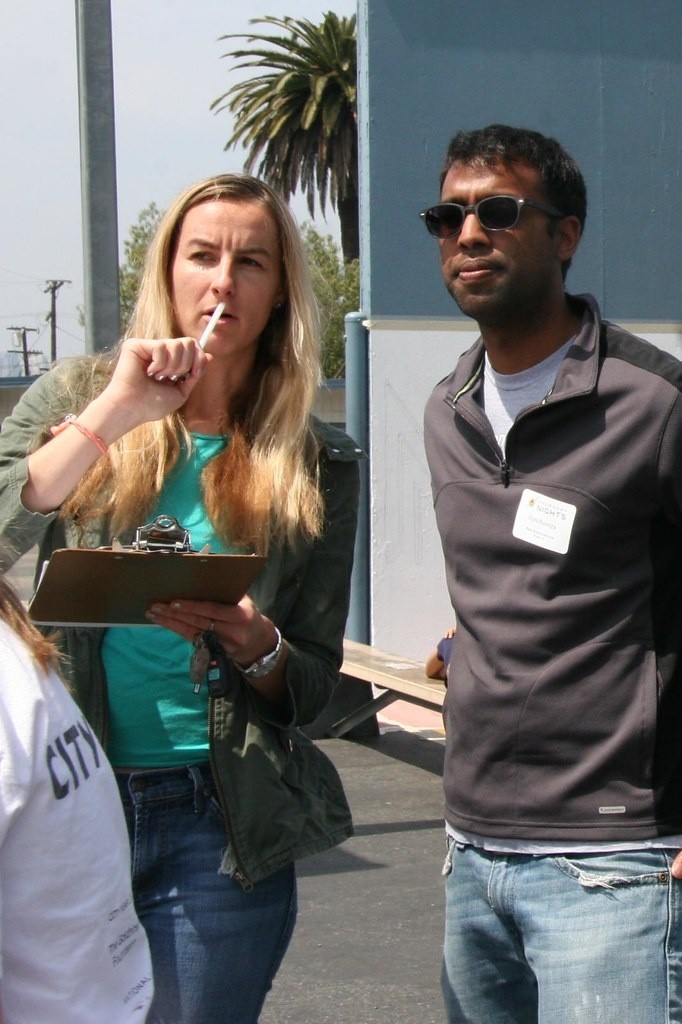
[[51, 413, 106, 454]]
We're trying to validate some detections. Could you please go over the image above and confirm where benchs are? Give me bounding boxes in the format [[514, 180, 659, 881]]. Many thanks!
[[330, 639, 447, 740]]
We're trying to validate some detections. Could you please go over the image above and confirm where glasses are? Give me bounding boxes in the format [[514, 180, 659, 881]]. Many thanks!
[[418, 195, 563, 240]]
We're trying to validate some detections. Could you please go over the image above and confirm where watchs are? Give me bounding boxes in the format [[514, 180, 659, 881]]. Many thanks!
[[235, 626, 283, 679]]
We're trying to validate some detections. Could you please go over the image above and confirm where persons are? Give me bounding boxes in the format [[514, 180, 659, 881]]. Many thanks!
[[1, 171, 361, 1024], [427, 629, 457, 680], [424, 125, 681, 1024], [1, 580, 154, 1024]]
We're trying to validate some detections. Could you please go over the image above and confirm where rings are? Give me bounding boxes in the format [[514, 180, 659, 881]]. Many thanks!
[[209, 619, 215, 631]]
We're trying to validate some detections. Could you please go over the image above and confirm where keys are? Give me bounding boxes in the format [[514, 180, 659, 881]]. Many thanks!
[[190, 631, 229, 699]]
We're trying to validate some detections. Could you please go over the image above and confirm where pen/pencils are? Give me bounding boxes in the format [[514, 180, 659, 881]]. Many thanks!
[[199, 302, 226, 349]]
